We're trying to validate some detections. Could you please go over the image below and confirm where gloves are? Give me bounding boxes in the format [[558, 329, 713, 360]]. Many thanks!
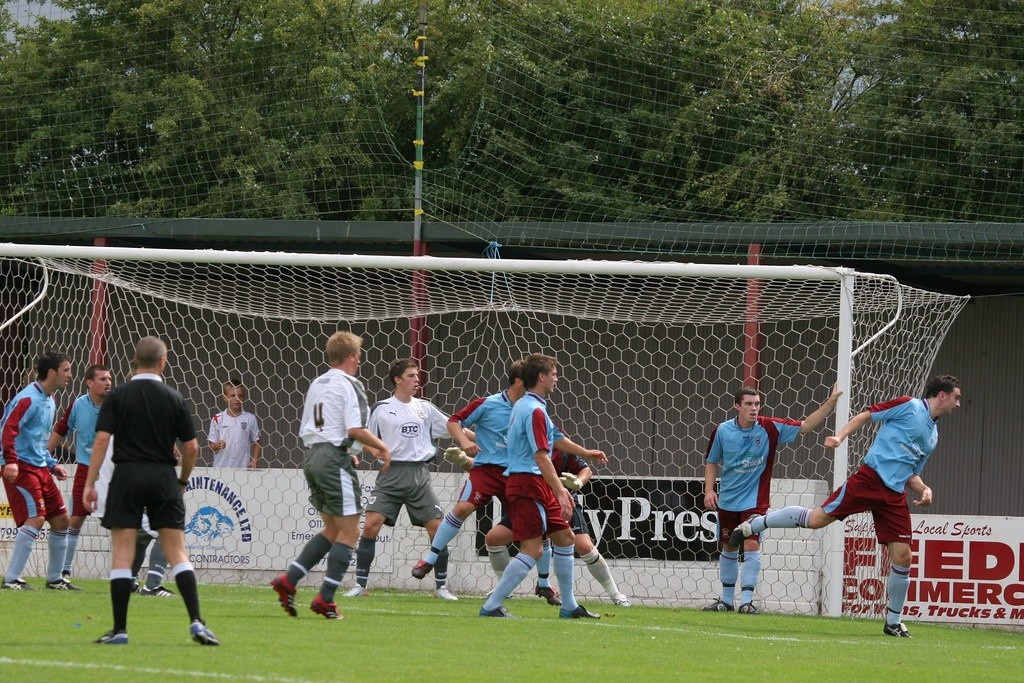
[[443, 446, 469, 467], [558, 472, 583, 492]]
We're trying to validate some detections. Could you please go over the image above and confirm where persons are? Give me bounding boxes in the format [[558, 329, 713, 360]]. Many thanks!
[[411, 353, 632, 619], [728, 375, 961, 637], [343, 358, 475, 600], [703, 384, 843, 614], [82, 335, 220, 645], [207, 380, 262, 468], [0, 350, 78, 590], [271, 331, 391, 620], [48, 364, 180, 598]]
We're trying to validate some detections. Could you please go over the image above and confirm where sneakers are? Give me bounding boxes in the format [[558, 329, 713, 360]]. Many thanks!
[[47, 570, 83, 590], [189, 619, 220, 645], [883, 620, 914, 639], [412, 559, 434, 580], [558, 604, 601, 619], [132, 576, 176, 598], [310, 594, 346, 619], [342, 583, 370, 597], [270, 572, 299, 617], [729, 514, 762, 548], [1, 578, 36, 591], [610, 594, 633, 607], [479, 606, 516, 618], [94, 630, 128, 643], [703, 600, 760, 614], [434, 584, 459, 601], [535, 581, 562, 606]]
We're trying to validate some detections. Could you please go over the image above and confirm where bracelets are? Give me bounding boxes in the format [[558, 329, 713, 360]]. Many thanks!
[[179, 480, 188, 485]]
